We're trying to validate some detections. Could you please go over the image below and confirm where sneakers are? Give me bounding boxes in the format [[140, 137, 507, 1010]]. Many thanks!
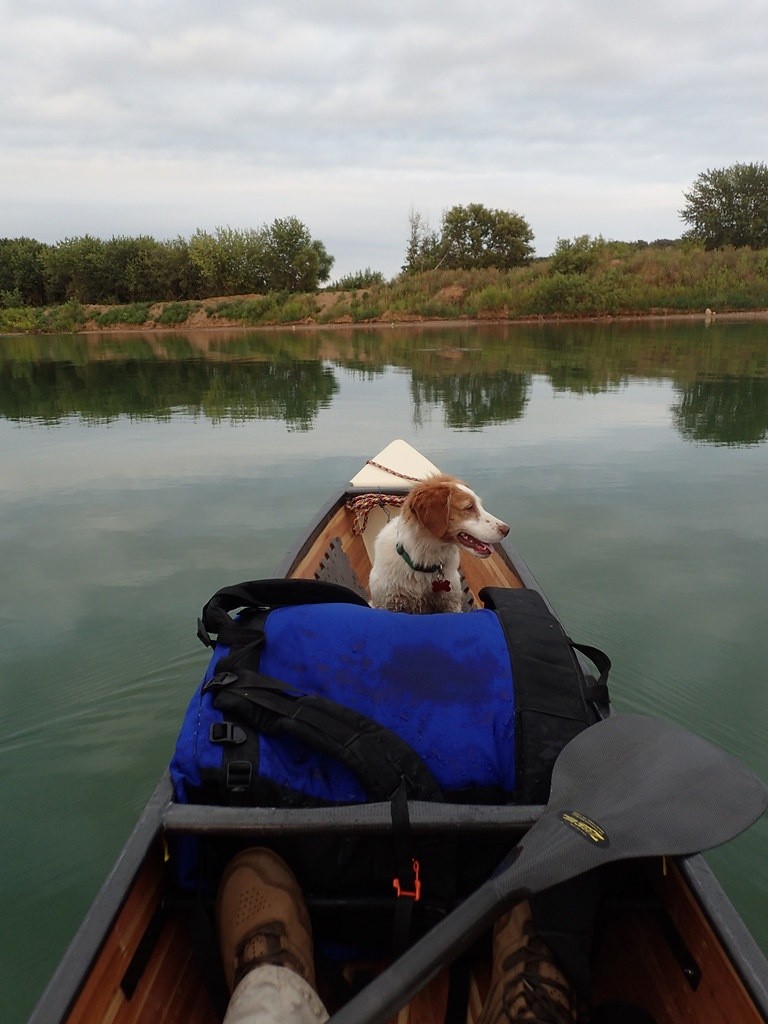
[[216, 848, 317, 998], [472, 894, 578, 1024]]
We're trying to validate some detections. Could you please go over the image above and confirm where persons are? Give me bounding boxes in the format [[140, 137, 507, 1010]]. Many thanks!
[[217, 847, 580, 1023]]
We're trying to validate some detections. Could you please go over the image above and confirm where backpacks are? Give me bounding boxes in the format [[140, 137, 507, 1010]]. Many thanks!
[[164, 575, 613, 921]]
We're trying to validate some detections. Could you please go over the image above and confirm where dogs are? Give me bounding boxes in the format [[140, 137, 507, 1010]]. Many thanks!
[[368, 473, 511, 613]]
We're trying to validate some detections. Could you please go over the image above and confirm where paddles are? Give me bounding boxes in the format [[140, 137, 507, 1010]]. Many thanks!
[[321, 711, 768, 1024]]
